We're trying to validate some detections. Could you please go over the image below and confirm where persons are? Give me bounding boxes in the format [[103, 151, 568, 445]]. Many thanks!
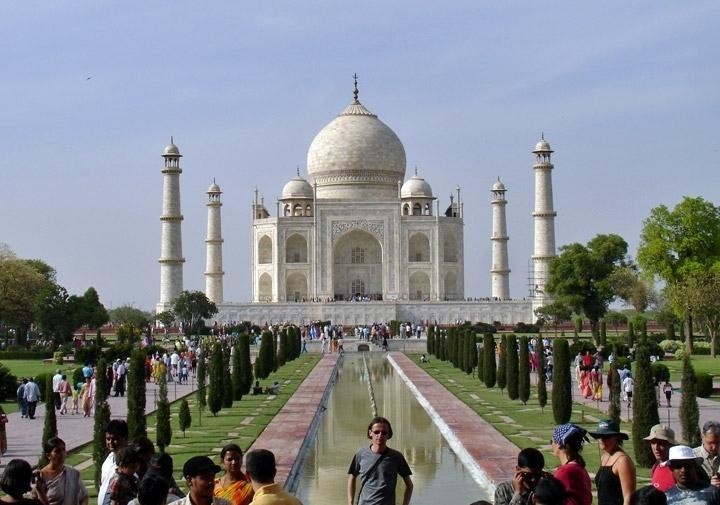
[[574, 351, 604, 402], [213, 444, 254, 505], [531, 478, 567, 505], [167, 456, 232, 505], [32, 437, 88, 505], [97, 419, 129, 505], [692, 421, 720, 487], [630, 485, 667, 505], [144, 333, 201, 385], [665, 445, 720, 505], [608, 352, 613, 370], [295, 294, 372, 302], [52, 368, 62, 409], [621, 364, 631, 401], [354, 322, 391, 348], [197, 320, 243, 347], [102, 437, 186, 505], [70, 386, 79, 415], [420, 354, 428, 363], [543, 337, 554, 381], [265, 381, 280, 395], [494, 447, 554, 505], [58, 374, 72, 415], [663, 380, 674, 408], [301, 324, 345, 353], [80, 377, 91, 417], [0, 459, 48, 505], [652, 376, 661, 407], [253, 384, 262, 394], [551, 423, 593, 505], [22, 376, 41, 419], [528, 337, 537, 371], [399, 320, 421, 340], [88, 378, 96, 415], [82, 363, 97, 381], [268, 321, 296, 334], [623, 373, 634, 407], [106, 357, 131, 397], [244, 449, 302, 505], [643, 424, 676, 493], [0, 405, 8, 457], [588, 420, 635, 505], [16, 378, 28, 418], [346, 416, 413, 505]]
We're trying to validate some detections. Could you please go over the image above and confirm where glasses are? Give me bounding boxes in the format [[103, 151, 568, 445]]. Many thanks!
[[373, 430, 388, 435], [522, 472, 542, 480]]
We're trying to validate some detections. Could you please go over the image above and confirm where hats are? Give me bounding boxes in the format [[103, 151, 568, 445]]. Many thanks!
[[587, 419, 629, 441], [659, 446, 704, 468], [643, 424, 675, 444], [183, 455, 221, 477]]
